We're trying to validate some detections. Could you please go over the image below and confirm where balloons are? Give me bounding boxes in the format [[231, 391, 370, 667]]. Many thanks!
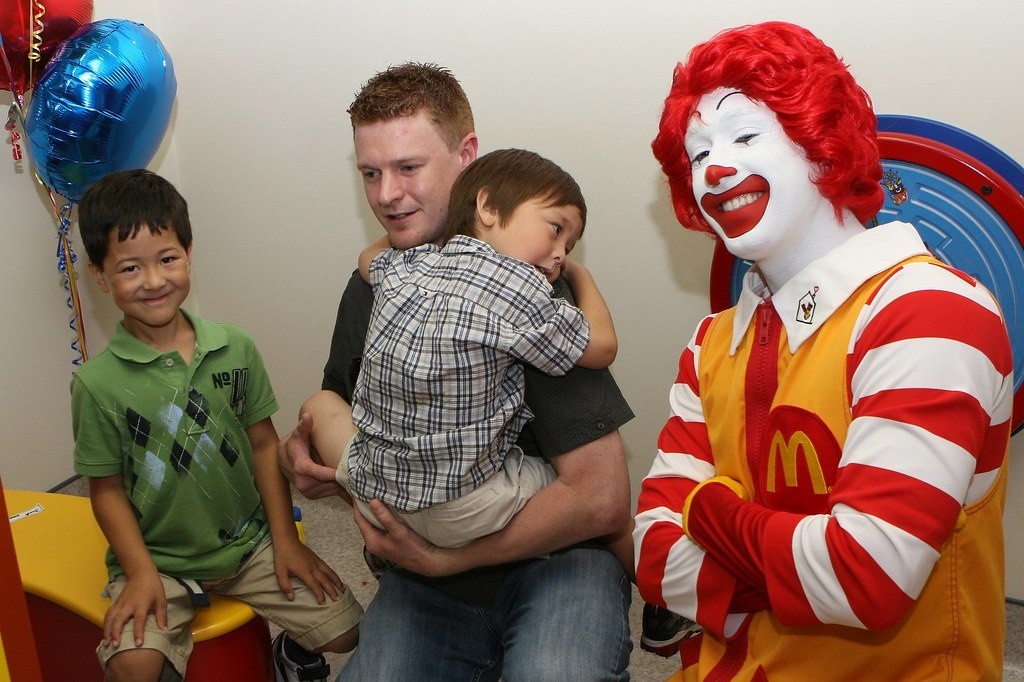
[[0, 0, 176, 204]]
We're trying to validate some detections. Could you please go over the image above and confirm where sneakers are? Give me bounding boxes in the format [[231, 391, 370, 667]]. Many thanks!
[[641, 597, 701, 658], [272, 628, 331, 682]]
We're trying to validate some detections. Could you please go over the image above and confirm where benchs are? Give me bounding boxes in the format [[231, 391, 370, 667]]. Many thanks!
[[2, 488, 277, 682]]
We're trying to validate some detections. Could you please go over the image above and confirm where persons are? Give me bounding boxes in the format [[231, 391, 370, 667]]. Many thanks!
[[298, 148, 705, 658], [632, 20, 1014, 682], [68, 168, 365, 682], [281, 61, 637, 682]]
